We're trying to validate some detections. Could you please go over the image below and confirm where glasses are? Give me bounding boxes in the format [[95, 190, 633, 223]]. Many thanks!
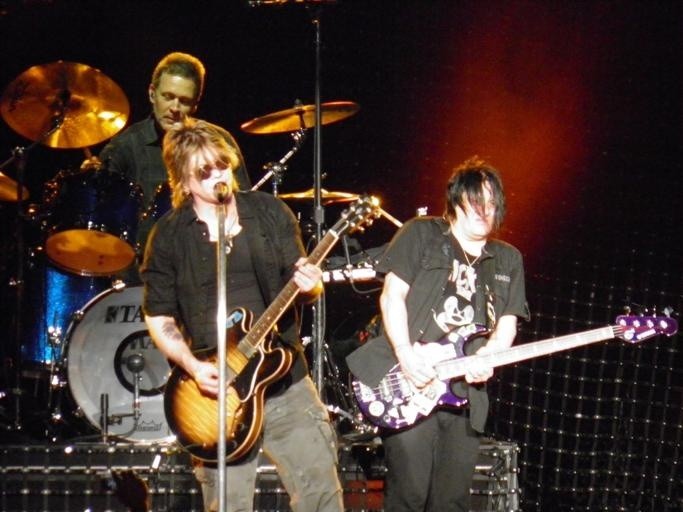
[[468, 198, 498, 209], [183, 153, 230, 182]]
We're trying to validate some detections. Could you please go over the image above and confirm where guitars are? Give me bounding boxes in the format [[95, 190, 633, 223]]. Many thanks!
[[163, 194, 383, 468], [350, 302, 679, 434]]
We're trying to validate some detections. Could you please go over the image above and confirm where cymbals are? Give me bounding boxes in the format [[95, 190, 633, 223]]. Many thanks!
[[240, 100, 361, 136], [276, 184, 361, 206], [0, 59, 132, 150]]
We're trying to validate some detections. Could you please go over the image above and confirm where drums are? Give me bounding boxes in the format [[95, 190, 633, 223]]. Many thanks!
[[18, 239, 139, 371], [57, 281, 181, 449], [47, 164, 147, 276], [147, 180, 173, 224]]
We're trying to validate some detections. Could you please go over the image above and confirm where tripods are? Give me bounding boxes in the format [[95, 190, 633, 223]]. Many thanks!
[[0, 123, 61, 439]]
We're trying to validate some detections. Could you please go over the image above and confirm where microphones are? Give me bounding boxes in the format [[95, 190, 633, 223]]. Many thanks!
[[213, 183, 229, 203]]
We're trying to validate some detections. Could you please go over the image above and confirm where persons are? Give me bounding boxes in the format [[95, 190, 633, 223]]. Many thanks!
[[377, 158, 529, 512], [143, 119, 343, 511], [99, 52, 250, 196]]
